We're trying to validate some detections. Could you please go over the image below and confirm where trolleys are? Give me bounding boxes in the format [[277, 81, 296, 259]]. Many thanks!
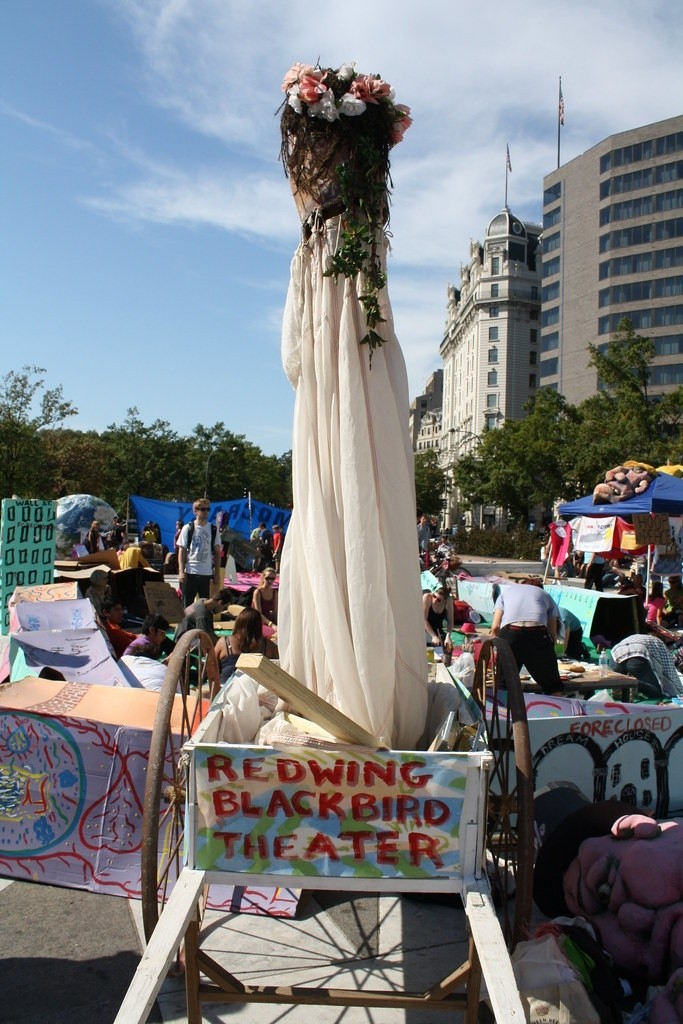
[[115, 629, 535, 1024]]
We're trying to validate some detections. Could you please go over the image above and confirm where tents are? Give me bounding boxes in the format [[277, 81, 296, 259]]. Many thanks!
[[559, 470, 683, 595]]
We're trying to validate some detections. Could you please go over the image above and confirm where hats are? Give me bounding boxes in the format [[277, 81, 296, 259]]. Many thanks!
[[668, 575, 680, 582]]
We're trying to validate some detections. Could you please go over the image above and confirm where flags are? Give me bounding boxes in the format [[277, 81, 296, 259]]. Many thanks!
[[558, 81, 564, 127], [507, 144, 511, 171]]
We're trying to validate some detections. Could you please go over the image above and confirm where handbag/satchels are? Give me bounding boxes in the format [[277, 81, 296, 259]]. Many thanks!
[[82, 533, 91, 551]]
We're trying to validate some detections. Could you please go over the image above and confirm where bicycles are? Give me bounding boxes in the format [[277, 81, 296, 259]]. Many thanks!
[[420, 552, 472, 599]]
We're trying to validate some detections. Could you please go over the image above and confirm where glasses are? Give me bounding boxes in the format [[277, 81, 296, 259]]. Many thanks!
[[264, 577, 276, 580], [433, 594, 441, 603], [197, 507, 211, 512], [669, 581, 677, 584]]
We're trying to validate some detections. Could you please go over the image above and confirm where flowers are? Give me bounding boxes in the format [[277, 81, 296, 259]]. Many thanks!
[[275, 59, 414, 360]]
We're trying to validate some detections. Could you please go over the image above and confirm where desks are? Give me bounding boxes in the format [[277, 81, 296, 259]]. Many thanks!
[[445, 577, 639, 640], [517, 656, 638, 704]]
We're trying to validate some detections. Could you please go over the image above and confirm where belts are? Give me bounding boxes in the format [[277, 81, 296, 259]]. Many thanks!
[[507, 624, 546, 632]]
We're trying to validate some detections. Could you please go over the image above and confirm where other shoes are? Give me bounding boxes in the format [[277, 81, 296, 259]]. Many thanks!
[[621, 685, 638, 703], [581, 641, 590, 659], [611, 686, 622, 701]]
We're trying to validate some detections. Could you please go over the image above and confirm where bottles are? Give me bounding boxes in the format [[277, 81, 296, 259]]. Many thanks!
[[426, 647, 435, 663], [599, 651, 607, 677]]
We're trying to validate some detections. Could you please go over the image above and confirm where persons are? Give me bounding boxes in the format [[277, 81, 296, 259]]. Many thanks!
[[124, 612, 207, 690], [175, 589, 235, 672], [102, 599, 143, 663], [422, 586, 454, 653], [88, 571, 145, 628], [494, 581, 565, 702], [416, 514, 459, 568], [176, 499, 222, 611], [541, 543, 632, 588], [617, 574, 683, 630], [584, 552, 606, 593], [254, 567, 278, 632], [610, 634, 683, 703], [207, 606, 279, 689], [554, 608, 583, 661], [250, 523, 285, 573], [67, 517, 185, 575]]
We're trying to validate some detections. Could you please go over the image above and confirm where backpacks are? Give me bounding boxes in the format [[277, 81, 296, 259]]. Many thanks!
[[264, 530, 274, 549]]
